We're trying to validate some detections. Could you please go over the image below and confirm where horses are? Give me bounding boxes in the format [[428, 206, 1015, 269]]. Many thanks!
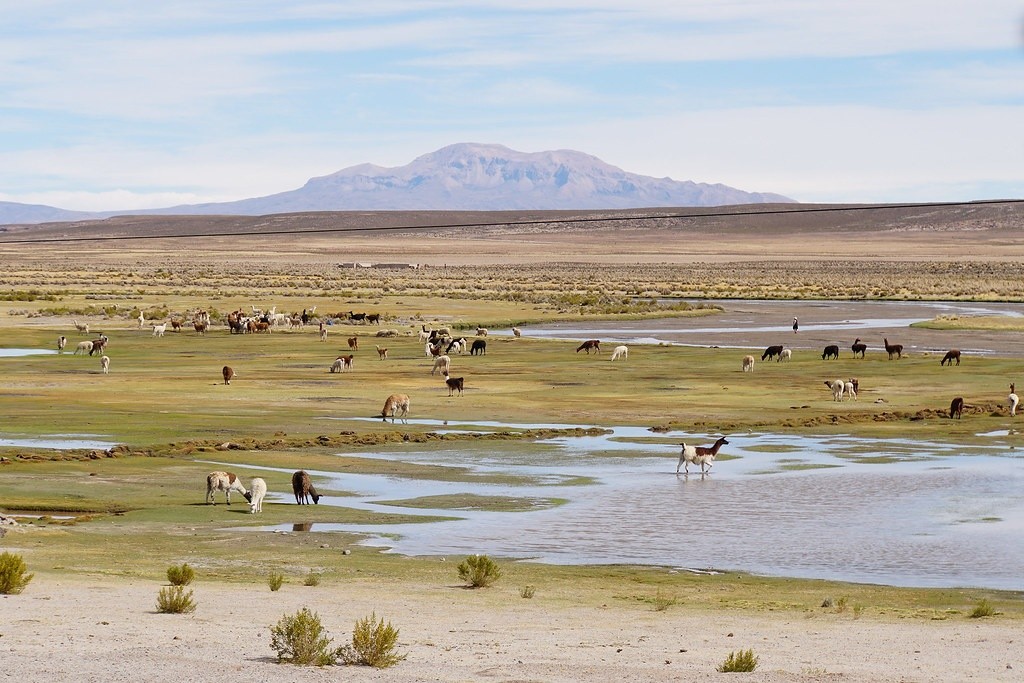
[[375, 345, 388, 360], [741, 345, 792, 372], [512, 327, 521, 338], [577, 340, 600, 355], [1008, 382, 1019, 417], [416, 324, 488, 397], [376, 328, 399, 338], [137, 311, 210, 337], [884, 337, 903, 360], [329, 354, 354, 374], [852, 338, 867, 360], [824, 377, 859, 402], [676, 436, 729, 474], [821, 345, 839, 360], [58, 319, 112, 374], [381, 393, 410, 417], [940, 349, 961, 367], [292, 470, 323, 507], [949, 397, 964, 419], [205, 470, 268, 513], [227, 304, 328, 343], [222, 366, 238, 386], [348, 310, 379, 324], [347, 336, 359, 352], [610, 345, 628, 362]]
